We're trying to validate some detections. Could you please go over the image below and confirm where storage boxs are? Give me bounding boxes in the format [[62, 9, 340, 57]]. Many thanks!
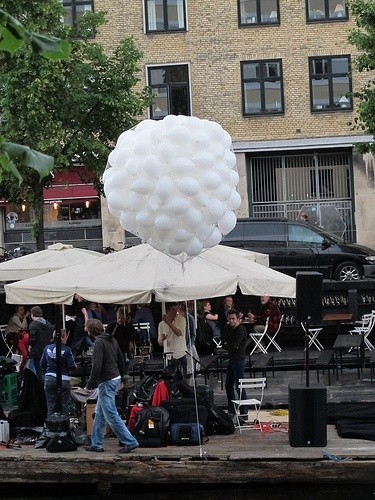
[[0, 373, 18, 407]]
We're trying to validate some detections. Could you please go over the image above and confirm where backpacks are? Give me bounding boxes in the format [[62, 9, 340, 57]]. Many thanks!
[[133, 406, 170, 447], [8, 409, 47, 428], [17, 368, 47, 412], [208, 406, 235, 435]]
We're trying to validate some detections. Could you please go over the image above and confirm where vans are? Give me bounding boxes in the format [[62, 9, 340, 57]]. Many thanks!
[[216, 215, 375, 281]]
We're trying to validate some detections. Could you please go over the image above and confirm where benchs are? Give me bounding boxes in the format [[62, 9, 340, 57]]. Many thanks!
[[251, 352, 273, 389], [317, 349, 335, 386], [369, 350, 375, 381]]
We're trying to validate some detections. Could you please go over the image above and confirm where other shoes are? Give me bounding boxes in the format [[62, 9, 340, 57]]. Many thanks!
[[254, 349, 262, 353], [227, 412, 235, 419], [118, 443, 139, 453], [85, 445, 104, 452], [239, 416, 248, 420]]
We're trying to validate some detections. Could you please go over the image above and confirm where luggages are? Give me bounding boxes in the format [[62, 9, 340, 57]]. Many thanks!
[[127, 406, 143, 435]]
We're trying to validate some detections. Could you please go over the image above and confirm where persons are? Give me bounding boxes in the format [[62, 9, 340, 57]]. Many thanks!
[[6, 295, 284, 453]]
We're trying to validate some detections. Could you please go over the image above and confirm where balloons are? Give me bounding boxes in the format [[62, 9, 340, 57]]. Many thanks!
[[103, 114, 241, 264]]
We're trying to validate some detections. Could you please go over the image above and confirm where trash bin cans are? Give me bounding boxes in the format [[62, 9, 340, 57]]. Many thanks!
[[86, 398, 108, 436]]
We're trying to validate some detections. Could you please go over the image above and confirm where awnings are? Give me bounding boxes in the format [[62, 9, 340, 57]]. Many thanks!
[[0, 166, 102, 204]]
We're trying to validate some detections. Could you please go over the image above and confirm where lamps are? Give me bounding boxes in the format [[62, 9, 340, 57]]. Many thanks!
[[339, 95, 350, 108], [270, 11, 277, 22], [334, 4, 344, 17]]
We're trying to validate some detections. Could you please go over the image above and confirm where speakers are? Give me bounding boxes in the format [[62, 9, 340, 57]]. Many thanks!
[[296, 271, 323, 322], [288, 383, 328, 447]]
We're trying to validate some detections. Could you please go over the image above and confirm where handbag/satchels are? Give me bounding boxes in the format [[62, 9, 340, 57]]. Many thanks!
[[161, 398, 196, 423], [46, 435, 77, 452], [171, 423, 203, 445]]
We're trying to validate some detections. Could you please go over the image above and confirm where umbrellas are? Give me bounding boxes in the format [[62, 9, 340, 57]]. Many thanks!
[[0, 244, 296, 332]]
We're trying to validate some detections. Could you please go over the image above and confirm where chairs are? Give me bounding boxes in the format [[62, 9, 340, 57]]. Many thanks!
[[204, 319, 227, 356], [348, 309, 375, 354], [249, 314, 284, 356], [301, 321, 324, 352], [132, 323, 153, 360], [231, 377, 266, 434]]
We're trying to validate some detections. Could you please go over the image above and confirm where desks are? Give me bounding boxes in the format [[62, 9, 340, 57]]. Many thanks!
[[227, 321, 257, 324], [323, 313, 352, 334], [333, 333, 364, 380]]
